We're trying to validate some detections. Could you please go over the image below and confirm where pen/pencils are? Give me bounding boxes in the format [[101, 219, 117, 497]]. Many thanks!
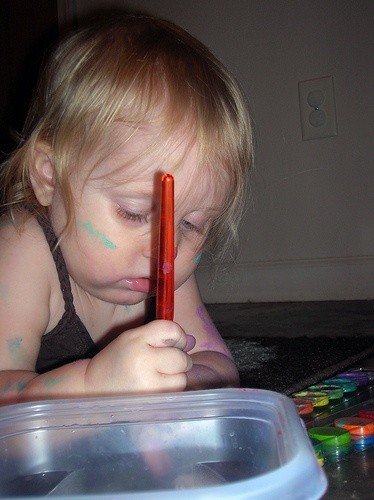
[[155, 174, 176, 320]]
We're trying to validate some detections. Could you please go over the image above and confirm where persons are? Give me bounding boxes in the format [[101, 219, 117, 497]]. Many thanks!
[[2, 11, 256, 392]]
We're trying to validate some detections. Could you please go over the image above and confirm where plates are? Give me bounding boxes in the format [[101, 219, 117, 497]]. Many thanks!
[[0, 392, 325, 500]]
